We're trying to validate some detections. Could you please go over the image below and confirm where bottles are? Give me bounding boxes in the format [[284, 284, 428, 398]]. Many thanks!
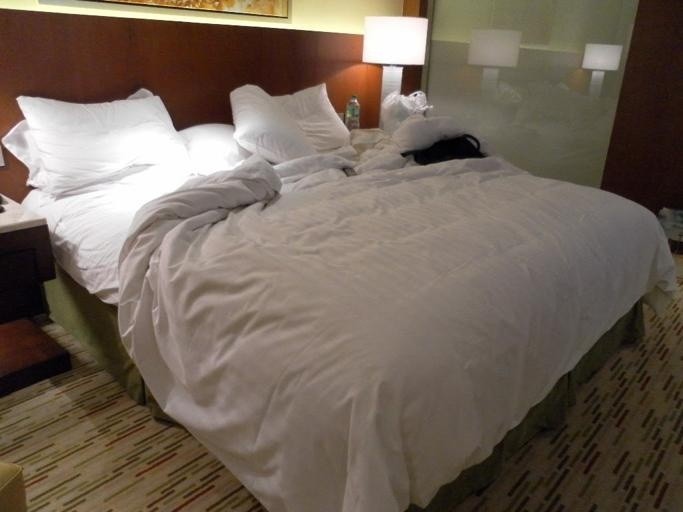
[[345, 94, 360, 130]]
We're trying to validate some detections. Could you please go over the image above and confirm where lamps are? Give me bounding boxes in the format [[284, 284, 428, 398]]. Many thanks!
[[360, 13, 430, 124], [464, 28, 524, 88], [580, 39, 625, 94]]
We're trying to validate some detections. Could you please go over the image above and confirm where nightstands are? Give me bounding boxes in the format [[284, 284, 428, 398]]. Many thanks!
[[0, 190, 62, 325]]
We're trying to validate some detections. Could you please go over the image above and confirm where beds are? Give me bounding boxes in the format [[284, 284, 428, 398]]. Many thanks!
[[0, 8, 677, 512]]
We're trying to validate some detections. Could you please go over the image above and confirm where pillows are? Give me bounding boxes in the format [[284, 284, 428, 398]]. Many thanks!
[[175, 120, 253, 176], [228, 77, 353, 164], [16, 94, 185, 194]]
